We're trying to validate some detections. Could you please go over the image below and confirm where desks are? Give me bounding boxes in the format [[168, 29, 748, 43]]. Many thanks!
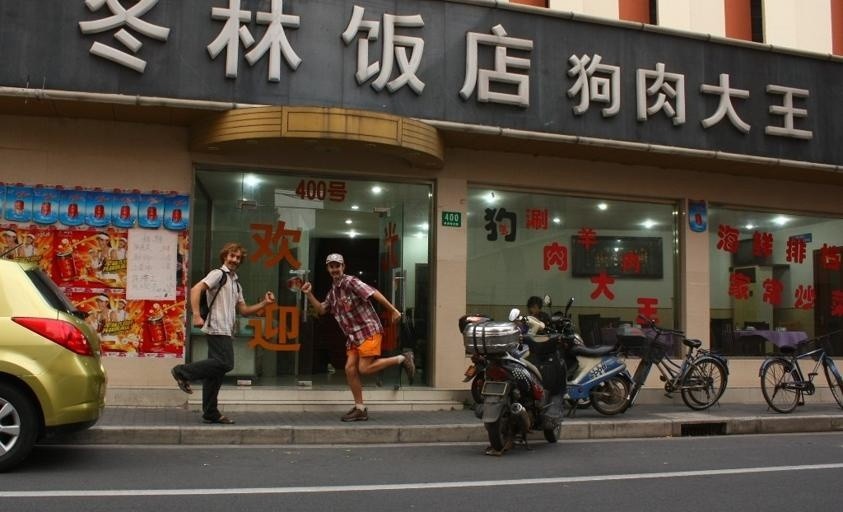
[[600, 327, 671, 357], [733, 330, 807, 355]]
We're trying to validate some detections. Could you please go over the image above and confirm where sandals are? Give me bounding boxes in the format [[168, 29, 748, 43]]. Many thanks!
[[200, 415, 234, 424], [171, 368, 193, 393]]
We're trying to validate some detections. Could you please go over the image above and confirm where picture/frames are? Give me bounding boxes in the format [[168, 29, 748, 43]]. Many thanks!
[[571, 235, 663, 278]]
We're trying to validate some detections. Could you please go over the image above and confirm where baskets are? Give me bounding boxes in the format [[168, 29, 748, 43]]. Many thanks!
[[808, 338, 833, 361], [639, 338, 668, 365]]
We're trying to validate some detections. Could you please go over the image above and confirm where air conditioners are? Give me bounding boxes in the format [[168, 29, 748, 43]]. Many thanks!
[[732, 265, 773, 353]]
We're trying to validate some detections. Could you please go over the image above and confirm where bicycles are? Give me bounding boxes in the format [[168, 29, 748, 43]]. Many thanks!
[[639, 316, 727, 403], [756, 330, 843, 415], [628, 324, 727, 410]]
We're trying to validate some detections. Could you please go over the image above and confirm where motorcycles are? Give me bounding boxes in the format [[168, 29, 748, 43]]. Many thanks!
[[451, 295, 574, 395], [463, 296, 576, 456], [561, 338, 632, 415]]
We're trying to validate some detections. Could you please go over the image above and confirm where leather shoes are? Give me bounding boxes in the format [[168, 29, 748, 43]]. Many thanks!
[[341, 407, 368, 422], [400, 352, 415, 385]]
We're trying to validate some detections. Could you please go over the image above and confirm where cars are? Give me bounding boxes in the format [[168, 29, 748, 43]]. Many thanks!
[[0, 258, 106, 471]]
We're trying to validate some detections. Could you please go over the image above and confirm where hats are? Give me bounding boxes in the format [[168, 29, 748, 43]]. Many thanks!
[[326, 253, 344, 264]]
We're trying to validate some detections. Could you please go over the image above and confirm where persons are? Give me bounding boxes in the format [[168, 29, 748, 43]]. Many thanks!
[[22, 232, 37, 257], [301, 252, 417, 421], [94, 293, 117, 323], [115, 236, 127, 260], [95, 232, 118, 261], [517, 295, 554, 335], [171, 240, 277, 424], [2, 229, 25, 257], [113, 297, 129, 322]]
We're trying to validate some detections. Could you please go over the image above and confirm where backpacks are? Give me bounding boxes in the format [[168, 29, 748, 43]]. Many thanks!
[[199, 269, 239, 320]]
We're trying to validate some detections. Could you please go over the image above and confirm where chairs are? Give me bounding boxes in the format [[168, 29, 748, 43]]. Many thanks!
[[711, 318, 736, 356], [743, 322, 769, 356], [578, 314, 601, 347], [612, 320, 634, 329], [591, 317, 620, 346]]
